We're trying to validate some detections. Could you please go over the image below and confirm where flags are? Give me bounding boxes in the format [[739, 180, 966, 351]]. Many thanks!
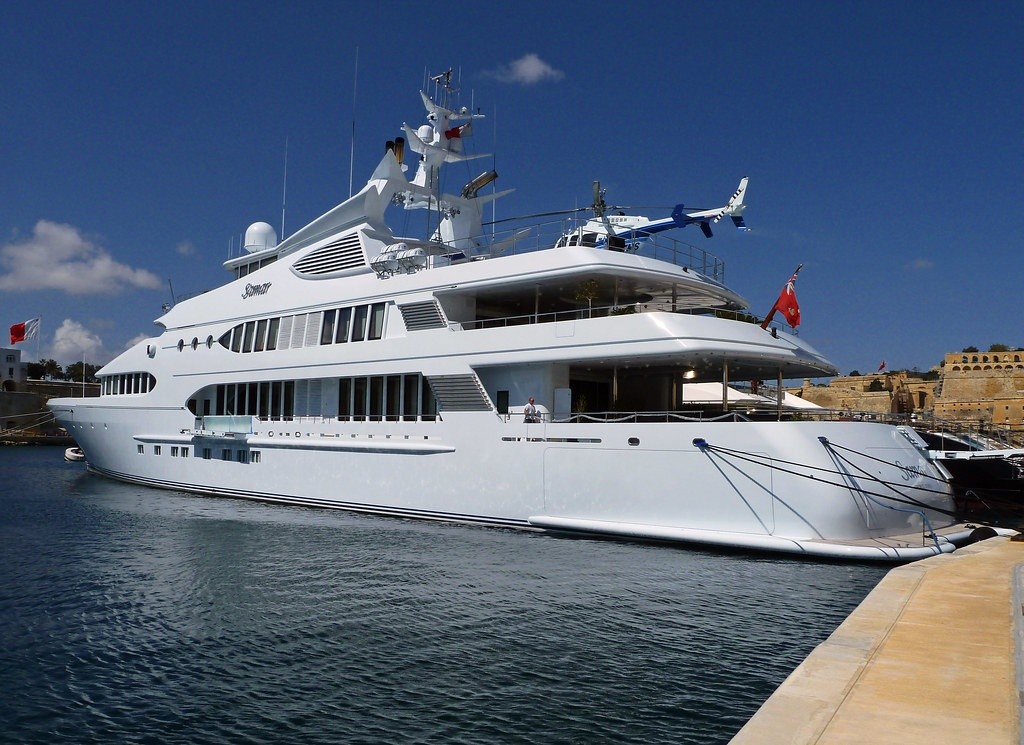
[[775, 272, 801, 329], [879, 360, 885, 371], [445, 122, 473, 140], [9, 318, 41, 345]]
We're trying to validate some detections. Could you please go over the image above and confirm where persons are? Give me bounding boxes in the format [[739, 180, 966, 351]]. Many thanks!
[[523, 397, 536, 422]]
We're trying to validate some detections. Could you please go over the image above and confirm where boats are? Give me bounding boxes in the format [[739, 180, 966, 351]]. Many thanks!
[[45, 40, 1024, 560]]
